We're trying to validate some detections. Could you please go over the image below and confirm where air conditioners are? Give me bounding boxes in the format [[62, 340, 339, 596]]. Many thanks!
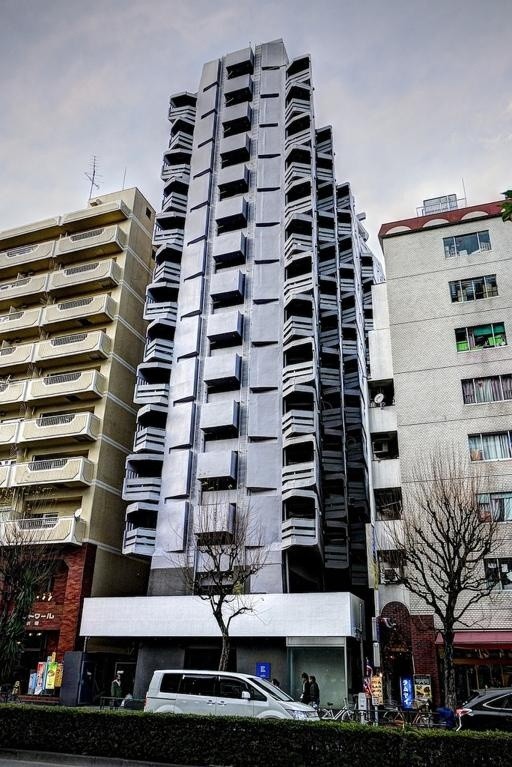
[[372, 441, 389, 453], [381, 567, 400, 583]]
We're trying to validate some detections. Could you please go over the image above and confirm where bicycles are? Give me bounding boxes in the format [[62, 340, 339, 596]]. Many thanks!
[[316, 697, 357, 724], [383, 700, 433, 733], [1, 684, 22, 704]]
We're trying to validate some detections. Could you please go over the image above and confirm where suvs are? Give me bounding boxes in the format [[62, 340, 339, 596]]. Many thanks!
[[452, 687, 512, 733]]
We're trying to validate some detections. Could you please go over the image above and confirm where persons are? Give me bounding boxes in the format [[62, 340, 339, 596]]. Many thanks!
[[272, 676, 279, 687], [308, 675, 321, 710], [299, 672, 311, 703], [11, 679, 21, 698], [110, 673, 123, 708]]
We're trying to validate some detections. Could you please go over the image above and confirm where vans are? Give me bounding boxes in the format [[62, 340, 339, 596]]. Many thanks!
[[144, 669, 321, 723]]
